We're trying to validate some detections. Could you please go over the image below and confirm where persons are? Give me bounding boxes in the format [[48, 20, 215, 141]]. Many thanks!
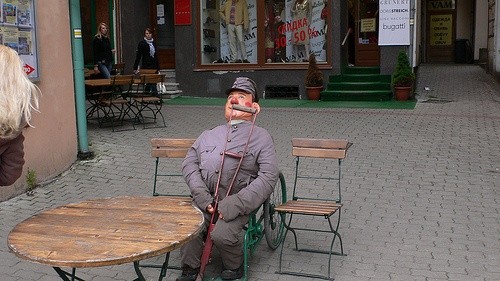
[[91, 22, 115, 101], [277, 23, 286, 62], [321, 2, 327, 24], [290, 0, 311, 62], [174, 76, 280, 281], [0, 43, 43, 189], [218, 0, 251, 63], [132, 27, 164, 104]]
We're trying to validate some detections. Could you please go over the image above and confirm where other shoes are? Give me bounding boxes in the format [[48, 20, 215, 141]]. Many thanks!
[[154, 99, 163, 104], [291, 58, 298, 62], [303, 58, 311, 62], [236, 60, 250, 63], [176, 264, 201, 281], [222, 260, 244, 280]]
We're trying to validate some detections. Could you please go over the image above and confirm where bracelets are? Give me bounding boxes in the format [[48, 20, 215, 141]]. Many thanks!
[[94, 65, 98, 67]]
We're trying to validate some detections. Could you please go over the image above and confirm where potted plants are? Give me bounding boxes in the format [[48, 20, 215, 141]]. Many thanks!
[[305, 52, 324, 100], [390, 49, 414, 101]]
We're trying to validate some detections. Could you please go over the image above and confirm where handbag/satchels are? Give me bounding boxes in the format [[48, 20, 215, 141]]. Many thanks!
[[156, 71, 166, 95]]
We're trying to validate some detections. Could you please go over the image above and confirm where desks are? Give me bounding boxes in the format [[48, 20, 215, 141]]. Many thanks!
[[7, 195, 207, 281], [85, 79, 142, 86]]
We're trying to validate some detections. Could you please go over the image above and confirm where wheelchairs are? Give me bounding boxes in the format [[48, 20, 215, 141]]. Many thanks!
[[207, 135, 287, 281]]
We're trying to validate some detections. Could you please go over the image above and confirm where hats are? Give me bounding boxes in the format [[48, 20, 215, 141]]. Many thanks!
[[224, 77, 259, 102]]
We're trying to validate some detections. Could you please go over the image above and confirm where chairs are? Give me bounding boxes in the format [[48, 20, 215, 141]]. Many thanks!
[[99, 63, 166, 132], [151, 138, 199, 198], [273, 138, 353, 281]]
